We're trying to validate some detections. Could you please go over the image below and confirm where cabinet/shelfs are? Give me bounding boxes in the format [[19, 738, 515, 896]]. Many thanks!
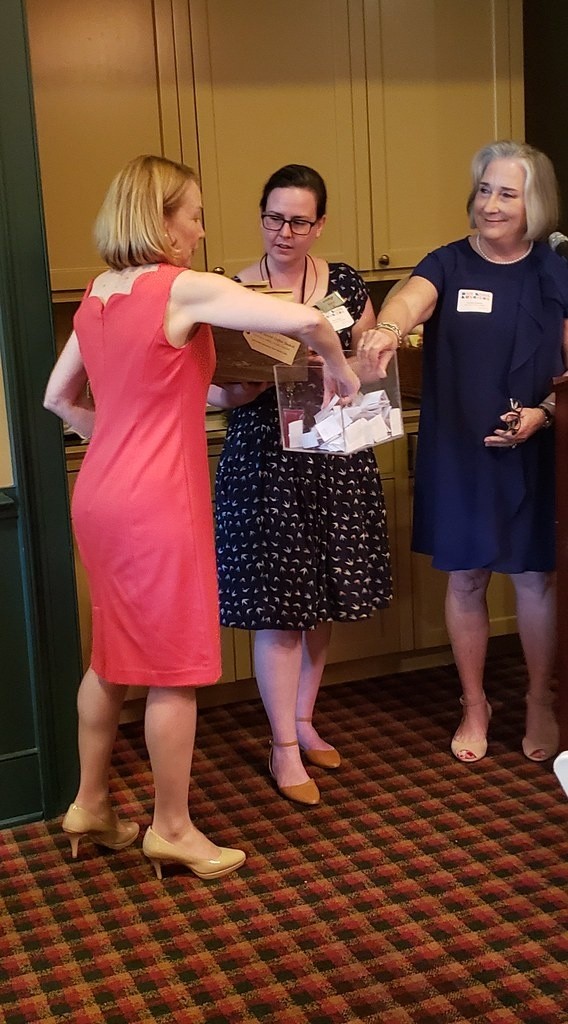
[[409, 419, 568, 669], [21, 0, 526, 303], [63, 423, 405, 727]]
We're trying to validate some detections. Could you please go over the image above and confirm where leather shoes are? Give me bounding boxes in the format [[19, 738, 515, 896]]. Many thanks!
[[269, 738, 320, 804], [295, 716, 340, 768]]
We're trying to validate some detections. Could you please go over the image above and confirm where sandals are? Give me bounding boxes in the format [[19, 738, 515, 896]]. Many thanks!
[[451, 690, 492, 762], [522, 691, 559, 761]]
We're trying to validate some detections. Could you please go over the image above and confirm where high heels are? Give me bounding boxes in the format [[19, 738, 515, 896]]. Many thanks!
[[62, 803, 140, 858], [142, 825, 246, 879]]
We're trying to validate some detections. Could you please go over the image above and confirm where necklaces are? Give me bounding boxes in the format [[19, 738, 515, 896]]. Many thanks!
[[476, 234, 533, 265], [259, 253, 319, 306]]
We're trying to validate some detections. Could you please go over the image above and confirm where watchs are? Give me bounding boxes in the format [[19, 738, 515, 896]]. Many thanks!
[[538, 405, 554, 429]]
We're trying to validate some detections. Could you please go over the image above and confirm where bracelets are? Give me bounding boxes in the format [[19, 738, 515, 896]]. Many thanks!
[[542, 400, 555, 406], [374, 322, 403, 348]]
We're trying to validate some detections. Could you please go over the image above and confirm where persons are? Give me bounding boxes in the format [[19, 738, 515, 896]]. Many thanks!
[[357, 140, 568, 764], [44, 156, 362, 879], [207, 164, 375, 805]]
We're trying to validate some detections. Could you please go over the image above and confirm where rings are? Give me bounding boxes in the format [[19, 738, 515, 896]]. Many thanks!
[[511, 443, 517, 449]]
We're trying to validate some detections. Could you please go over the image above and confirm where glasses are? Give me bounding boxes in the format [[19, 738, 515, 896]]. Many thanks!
[[506, 398, 523, 435], [261, 214, 317, 235]]
